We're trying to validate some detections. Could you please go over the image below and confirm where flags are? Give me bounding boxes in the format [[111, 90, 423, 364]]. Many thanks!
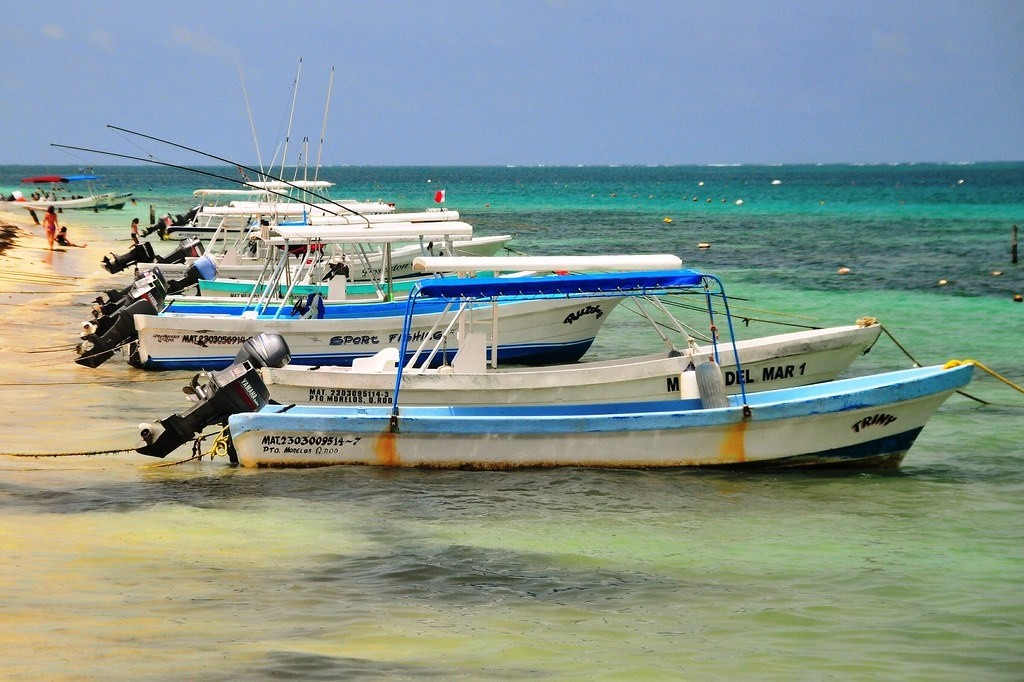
[[435, 190, 445, 203]]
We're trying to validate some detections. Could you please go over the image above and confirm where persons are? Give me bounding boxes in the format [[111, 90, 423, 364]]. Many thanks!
[[31, 186, 83, 201], [42, 206, 58, 249], [131, 199, 135, 204], [129, 218, 139, 249], [55, 227, 87, 248], [57, 207, 62, 213]]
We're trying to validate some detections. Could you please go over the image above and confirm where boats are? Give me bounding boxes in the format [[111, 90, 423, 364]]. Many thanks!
[[98, 180, 522, 285], [74, 296, 632, 369], [186, 250, 892, 403], [134, 270, 983, 472], [0, 172, 135, 211]]
